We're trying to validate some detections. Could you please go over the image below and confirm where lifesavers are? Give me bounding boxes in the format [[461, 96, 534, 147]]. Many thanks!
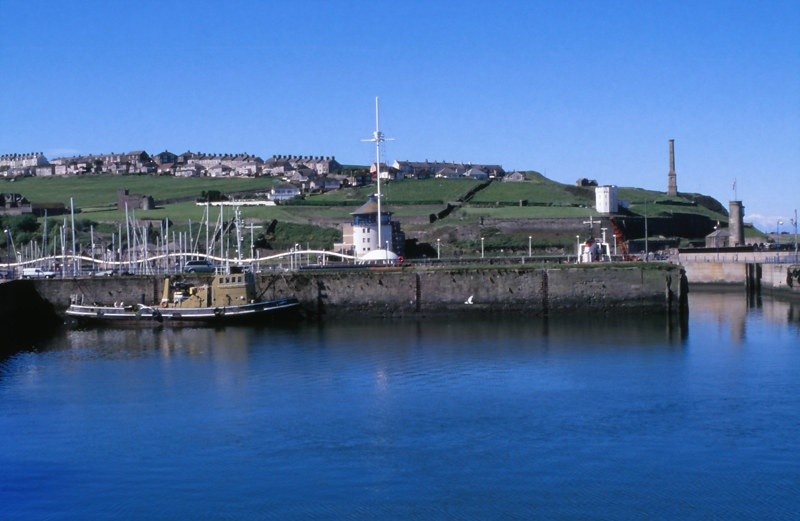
[[160, 301, 168, 308]]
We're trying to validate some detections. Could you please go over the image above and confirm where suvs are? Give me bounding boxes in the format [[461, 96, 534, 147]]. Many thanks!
[[23, 268, 56, 279], [184, 259, 223, 273]]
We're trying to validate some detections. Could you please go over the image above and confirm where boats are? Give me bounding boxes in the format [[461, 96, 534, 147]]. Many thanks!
[[65, 294, 136, 319], [139, 251, 301, 317]]
